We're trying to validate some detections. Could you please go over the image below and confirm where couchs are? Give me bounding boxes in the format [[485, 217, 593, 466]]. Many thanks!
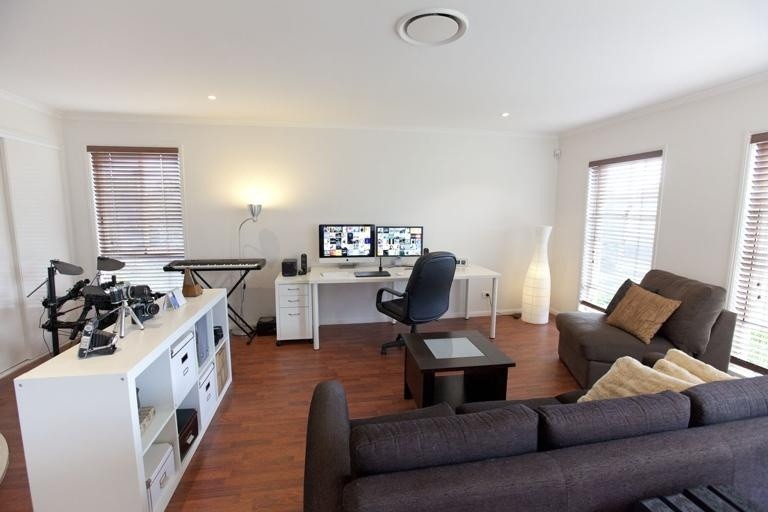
[[303, 379, 768, 512]]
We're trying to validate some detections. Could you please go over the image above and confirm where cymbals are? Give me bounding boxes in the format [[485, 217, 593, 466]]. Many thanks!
[[49, 260, 84, 275], [97, 256, 125, 271]]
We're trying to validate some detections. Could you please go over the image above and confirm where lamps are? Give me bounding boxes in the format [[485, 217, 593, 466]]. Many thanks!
[[238, 204, 261, 258]]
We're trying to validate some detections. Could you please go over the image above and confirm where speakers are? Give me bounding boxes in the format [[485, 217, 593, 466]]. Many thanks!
[[256, 316, 276, 336], [282, 258, 297, 276]]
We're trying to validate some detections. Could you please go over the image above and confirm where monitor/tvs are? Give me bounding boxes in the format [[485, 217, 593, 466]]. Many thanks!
[[375, 225, 423, 267], [318, 224, 376, 269]]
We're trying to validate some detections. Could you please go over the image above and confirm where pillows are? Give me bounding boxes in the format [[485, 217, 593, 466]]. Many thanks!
[[578, 355, 695, 403], [348, 403, 538, 477], [665, 348, 739, 385], [606, 283, 682, 345], [680, 377, 768, 428], [640, 269, 727, 358], [652, 359, 705, 396], [534, 390, 692, 452], [604, 278, 659, 316]]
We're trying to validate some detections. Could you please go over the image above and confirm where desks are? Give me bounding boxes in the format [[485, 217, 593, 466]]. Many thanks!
[[308, 265, 501, 350]]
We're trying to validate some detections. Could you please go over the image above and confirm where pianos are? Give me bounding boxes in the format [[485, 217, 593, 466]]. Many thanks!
[[163, 258, 266, 272]]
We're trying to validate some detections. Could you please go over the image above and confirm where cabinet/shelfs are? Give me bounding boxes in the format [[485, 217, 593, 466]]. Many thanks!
[[274, 272, 312, 346], [13, 287, 233, 512]]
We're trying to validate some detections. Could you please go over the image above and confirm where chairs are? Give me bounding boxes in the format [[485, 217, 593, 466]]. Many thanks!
[[556, 308, 737, 390], [376, 251, 456, 355]]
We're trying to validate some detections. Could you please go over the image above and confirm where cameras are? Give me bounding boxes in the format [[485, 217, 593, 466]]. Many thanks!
[[132, 297, 158, 324], [77, 322, 116, 358]]
